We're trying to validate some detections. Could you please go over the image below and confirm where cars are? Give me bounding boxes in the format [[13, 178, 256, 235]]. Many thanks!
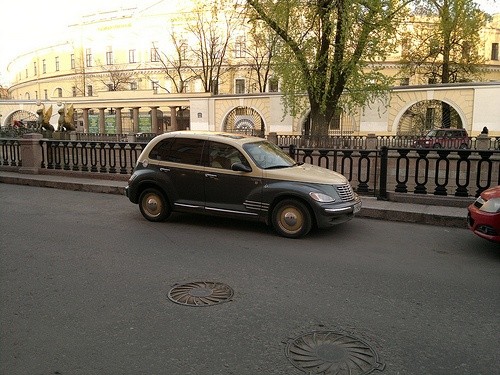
[[126, 130, 363, 239], [467, 185, 500, 243]]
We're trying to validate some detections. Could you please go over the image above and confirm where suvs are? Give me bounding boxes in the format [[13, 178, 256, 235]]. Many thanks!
[[413, 129, 470, 148]]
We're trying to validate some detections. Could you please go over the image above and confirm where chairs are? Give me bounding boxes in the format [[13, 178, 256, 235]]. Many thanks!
[[211, 154, 231, 168], [229, 156, 243, 169]]
